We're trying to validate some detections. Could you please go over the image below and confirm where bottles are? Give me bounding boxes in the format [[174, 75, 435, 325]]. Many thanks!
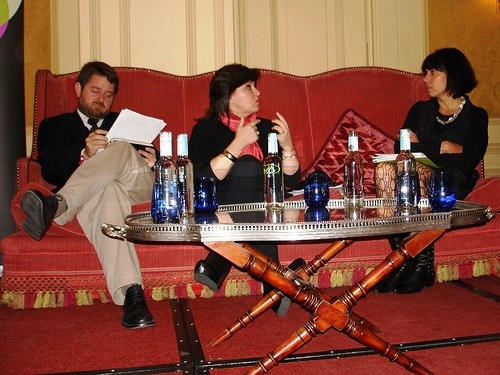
[[343, 130, 365, 210], [344, 210, 365, 219], [395, 129, 416, 212], [175, 133, 196, 219], [265, 212, 283, 222], [151, 131, 180, 223], [263, 132, 285, 212]]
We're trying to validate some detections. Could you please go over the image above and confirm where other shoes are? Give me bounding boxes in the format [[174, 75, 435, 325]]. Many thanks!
[[195, 248, 231, 291], [265, 257, 306, 319]]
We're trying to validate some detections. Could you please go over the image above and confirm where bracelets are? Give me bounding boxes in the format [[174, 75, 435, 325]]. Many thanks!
[[282, 150, 296, 161], [222, 148, 238, 163]]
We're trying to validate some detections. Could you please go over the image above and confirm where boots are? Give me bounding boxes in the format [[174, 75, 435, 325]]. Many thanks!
[[371, 233, 411, 293], [393, 241, 436, 293]]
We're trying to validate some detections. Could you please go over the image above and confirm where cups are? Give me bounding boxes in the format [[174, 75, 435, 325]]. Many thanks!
[[194, 176, 218, 213], [303, 174, 329, 208], [398, 170, 420, 212], [427, 170, 457, 211], [195, 213, 219, 224], [305, 208, 329, 221]]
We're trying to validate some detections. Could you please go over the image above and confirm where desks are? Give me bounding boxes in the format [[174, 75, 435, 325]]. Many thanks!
[[99, 196, 496, 375]]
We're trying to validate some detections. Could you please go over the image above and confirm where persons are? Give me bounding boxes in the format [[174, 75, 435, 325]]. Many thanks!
[[187, 63, 307, 317], [368, 48, 489, 295], [21, 61, 159, 330]]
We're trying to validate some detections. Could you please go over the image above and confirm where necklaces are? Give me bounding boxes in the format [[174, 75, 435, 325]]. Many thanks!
[[433, 96, 466, 125]]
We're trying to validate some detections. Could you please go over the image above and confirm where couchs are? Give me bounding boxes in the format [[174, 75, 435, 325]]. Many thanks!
[[1, 67, 500, 310]]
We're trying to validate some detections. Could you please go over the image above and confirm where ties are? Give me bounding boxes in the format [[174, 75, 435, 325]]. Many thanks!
[[86, 118, 101, 135]]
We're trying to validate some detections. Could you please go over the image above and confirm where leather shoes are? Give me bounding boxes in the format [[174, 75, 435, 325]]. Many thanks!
[[20, 190, 63, 242], [120, 283, 156, 329]]
